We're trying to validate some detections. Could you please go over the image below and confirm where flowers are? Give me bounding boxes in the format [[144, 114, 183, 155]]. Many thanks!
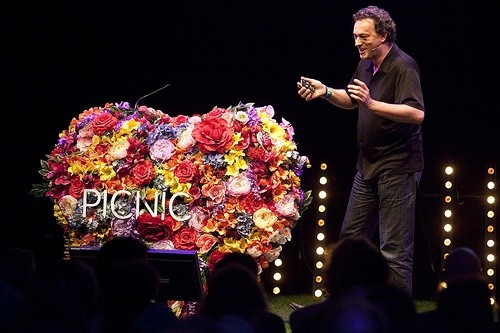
[[31, 101, 313, 318]]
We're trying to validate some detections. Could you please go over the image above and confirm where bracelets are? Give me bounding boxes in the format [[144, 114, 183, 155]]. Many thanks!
[[322, 87, 332, 99]]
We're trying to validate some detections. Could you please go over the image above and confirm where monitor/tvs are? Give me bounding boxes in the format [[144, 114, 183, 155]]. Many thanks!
[[146, 246, 202, 303]]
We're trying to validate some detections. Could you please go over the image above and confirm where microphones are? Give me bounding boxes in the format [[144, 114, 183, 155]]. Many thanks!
[[371, 41, 386, 51]]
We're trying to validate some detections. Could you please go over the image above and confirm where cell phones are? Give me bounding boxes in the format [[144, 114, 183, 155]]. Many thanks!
[[300, 79, 315, 93]]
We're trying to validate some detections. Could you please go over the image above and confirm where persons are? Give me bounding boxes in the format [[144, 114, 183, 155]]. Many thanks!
[[0, 222, 494, 333], [297, 5, 425, 297]]
